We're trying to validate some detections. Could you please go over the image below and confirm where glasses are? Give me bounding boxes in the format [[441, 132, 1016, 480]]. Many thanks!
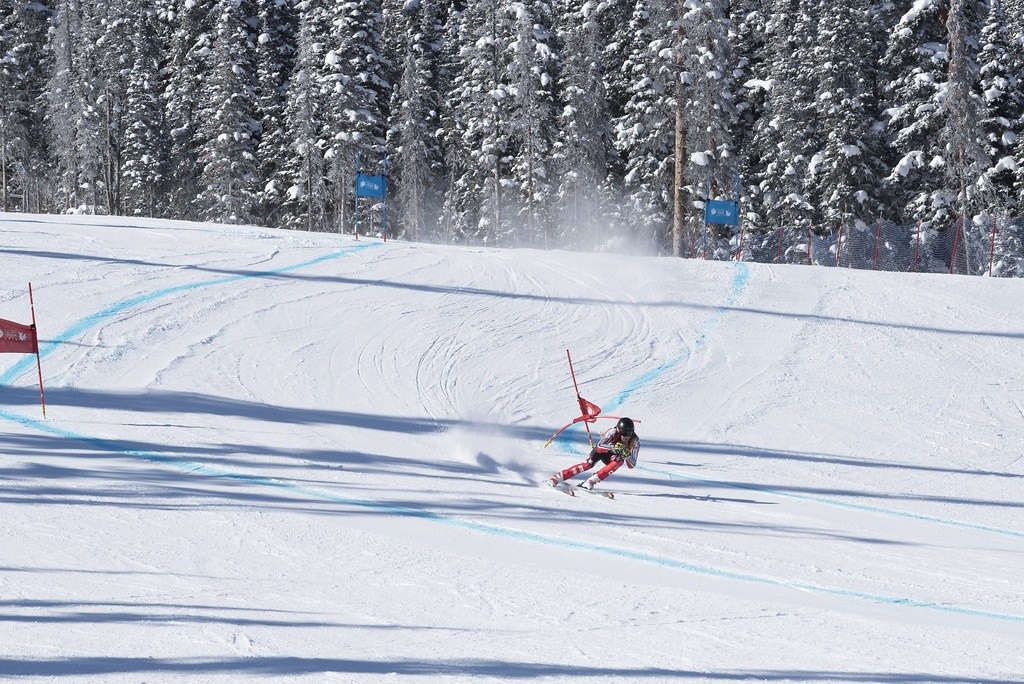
[[620, 431, 633, 437]]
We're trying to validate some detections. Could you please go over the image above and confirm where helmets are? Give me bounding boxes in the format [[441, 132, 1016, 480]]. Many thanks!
[[616, 417, 634, 434]]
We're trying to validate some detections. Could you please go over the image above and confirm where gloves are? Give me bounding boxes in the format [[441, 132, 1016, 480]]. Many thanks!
[[613, 442, 625, 454], [620, 444, 631, 457]]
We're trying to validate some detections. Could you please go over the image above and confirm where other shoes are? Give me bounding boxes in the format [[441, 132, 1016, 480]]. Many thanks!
[[547, 476, 557, 487], [581, 480, 593, 490]]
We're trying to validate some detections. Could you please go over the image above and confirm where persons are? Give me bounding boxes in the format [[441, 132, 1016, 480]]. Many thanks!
[[548, 417, 641, 491]]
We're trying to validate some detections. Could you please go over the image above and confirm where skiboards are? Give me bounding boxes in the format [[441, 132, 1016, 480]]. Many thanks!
[[544, 480, 615, 500]]
